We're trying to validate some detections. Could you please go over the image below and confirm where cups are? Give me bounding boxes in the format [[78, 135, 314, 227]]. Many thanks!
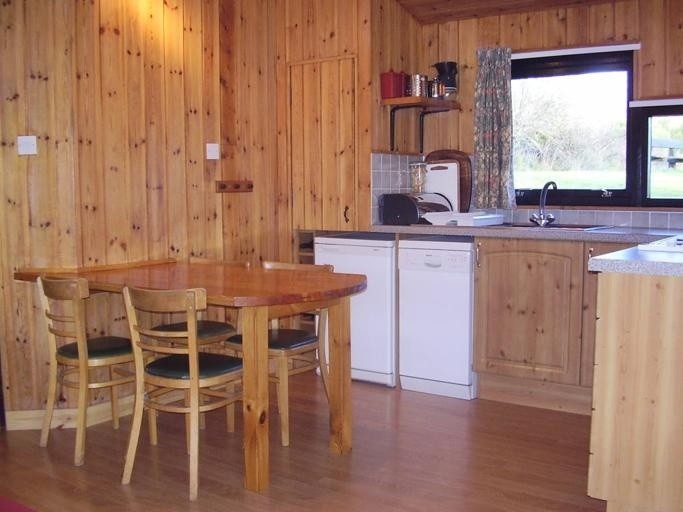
[[410, 160, 425, 194], [433, 61, 457, 86], [379, 73, 410, 98]]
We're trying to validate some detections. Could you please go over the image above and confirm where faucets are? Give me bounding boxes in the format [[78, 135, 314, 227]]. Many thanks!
[[530, 181, 557, 227]]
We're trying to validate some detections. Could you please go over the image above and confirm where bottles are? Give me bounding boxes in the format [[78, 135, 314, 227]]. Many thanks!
[[409, 74, 428, 96], [428, 77, 446, 98], [444, 87, 459, 101]]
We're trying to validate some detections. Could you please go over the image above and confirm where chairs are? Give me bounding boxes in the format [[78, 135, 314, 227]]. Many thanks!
[[223, 262, 334, 446], [147, 257, 250, 433], [36, 277, 157, 467], [121, 282, 242, 502]]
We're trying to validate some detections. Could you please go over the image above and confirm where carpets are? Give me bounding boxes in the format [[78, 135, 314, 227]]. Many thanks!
[[0, 495, 36, 512]]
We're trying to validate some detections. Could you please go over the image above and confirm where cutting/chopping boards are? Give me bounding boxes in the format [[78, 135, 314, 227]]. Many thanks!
[[425, 150, 471, 214]]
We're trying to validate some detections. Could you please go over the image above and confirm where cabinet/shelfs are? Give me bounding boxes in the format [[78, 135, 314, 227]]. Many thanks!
[[471, 236, 584, 412], [578, 242, 639, 414]]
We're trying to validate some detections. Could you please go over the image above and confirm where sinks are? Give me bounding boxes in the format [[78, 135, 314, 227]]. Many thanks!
[[489, 223, 614, 231]]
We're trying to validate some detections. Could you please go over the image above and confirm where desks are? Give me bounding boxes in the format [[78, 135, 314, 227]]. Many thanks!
[[13, 258, 367, 492]]
